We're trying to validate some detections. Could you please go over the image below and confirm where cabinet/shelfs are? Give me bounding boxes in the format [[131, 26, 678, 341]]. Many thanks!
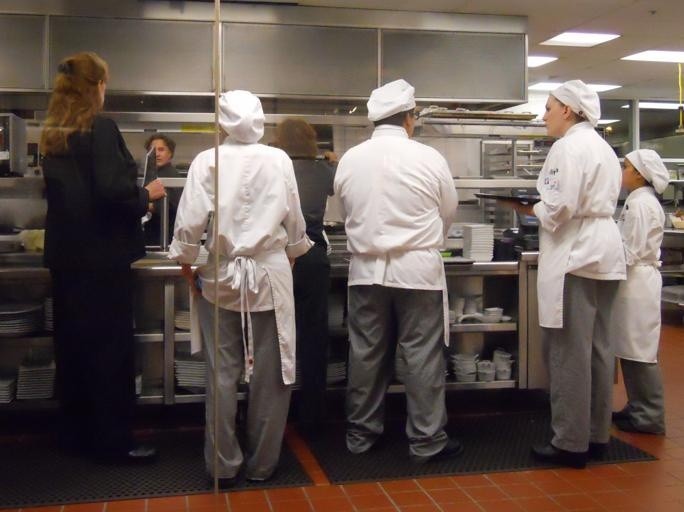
[[129, 265, 525, 402]]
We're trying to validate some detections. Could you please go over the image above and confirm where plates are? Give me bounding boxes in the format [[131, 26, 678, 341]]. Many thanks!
[[194, 246, 207, 268], [0, 294, 58, 408], [461, 223, 494, 262], [325, 288, 348, 393], [173, 303, 209, 394]]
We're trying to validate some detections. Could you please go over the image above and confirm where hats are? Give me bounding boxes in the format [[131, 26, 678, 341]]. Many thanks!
[[550, 79, 601, 127], [217, 90, 265, 144], [625, 148, 670, 194], [366, 79, 416, 122]]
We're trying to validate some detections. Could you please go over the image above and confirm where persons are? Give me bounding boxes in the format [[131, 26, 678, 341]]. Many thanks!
[[611, 145, 671, 436], [36, 49, 167, 465], [140, 131, 186, 249], [494, 77, 628, 470], [333, 77, 468, 466], [265, 114, 340, 445], [168, 88, 317, 487]]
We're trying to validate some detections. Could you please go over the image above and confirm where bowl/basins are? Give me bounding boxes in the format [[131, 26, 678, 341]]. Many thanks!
[[666, 212, 683, 229], [0, 235, 21, 252]]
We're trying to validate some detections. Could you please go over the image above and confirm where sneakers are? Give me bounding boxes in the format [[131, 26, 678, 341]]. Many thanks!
[[612, 411, 665, 433]]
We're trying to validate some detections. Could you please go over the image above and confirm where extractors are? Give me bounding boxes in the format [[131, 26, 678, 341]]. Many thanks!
[[0, 10, 528, 117]]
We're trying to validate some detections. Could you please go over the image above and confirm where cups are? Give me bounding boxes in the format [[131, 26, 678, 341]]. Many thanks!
[[450, 292, 511, 381]]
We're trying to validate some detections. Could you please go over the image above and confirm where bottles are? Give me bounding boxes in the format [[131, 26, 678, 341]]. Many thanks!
[[500, 227, 514, 260]]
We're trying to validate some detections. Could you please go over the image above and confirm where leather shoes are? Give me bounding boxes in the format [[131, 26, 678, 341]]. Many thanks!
[[587, 441, 609, 462], [208, 469, 239, 487], [348, 429, 389, 453], [58, 432, 159, 466], [529, 440, 586, 469], [409, 437, 464, 462]]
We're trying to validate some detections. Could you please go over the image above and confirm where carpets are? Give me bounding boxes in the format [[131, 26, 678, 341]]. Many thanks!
[[291, 406, 658, 486], [0, 424, 315, 506]]
